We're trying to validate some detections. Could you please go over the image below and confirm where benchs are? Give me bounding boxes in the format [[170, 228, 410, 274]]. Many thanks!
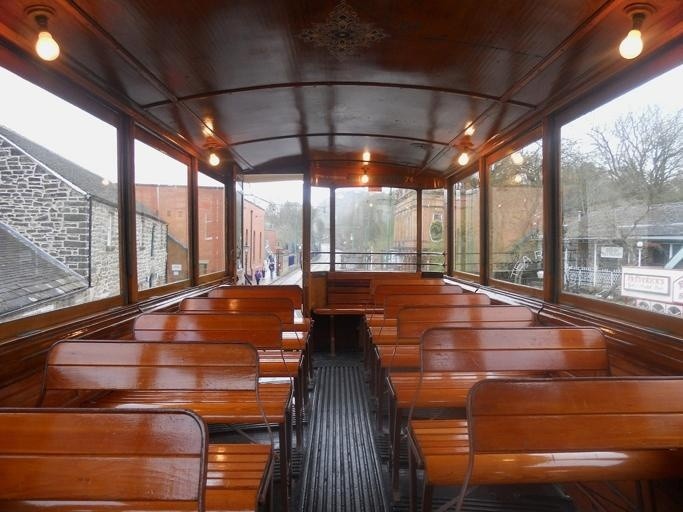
[[0, 270, 683, 512]]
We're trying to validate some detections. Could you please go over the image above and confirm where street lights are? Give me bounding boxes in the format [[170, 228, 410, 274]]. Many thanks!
[[636, 241, 644, 268]]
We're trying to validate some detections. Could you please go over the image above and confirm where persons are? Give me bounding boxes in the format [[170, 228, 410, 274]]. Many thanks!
[[261, 265, 266, 278], [254, 270, 261, 284]]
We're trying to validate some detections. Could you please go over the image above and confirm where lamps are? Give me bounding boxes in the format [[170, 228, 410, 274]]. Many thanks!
[[24, 5, 60, 62], [204, 143, 220, 166], [619, 3, 657, 59], [457, 143, 473, 166], [463, 121, 475, 143], [361, 166, 370, 184]]
